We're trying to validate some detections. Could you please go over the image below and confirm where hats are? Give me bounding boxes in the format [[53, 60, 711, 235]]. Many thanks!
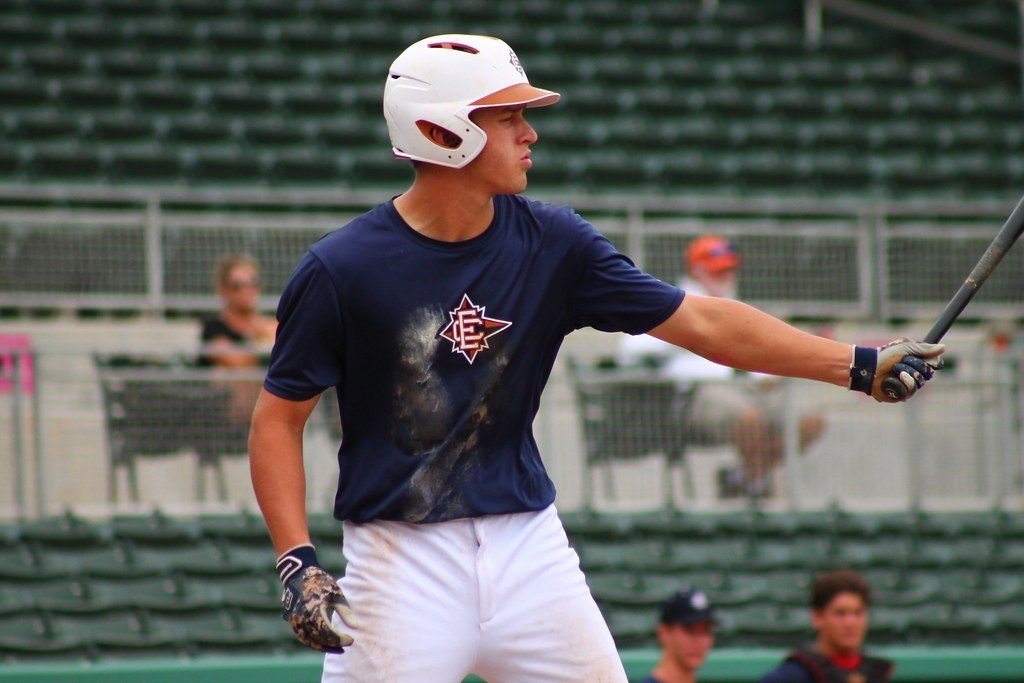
[[684, 233, 743, 272], [658, 586, 722, 625]]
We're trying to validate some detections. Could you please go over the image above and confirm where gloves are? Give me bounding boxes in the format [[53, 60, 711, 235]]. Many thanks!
[[276, 545, 357, 653], [850, 338, 945, 403]]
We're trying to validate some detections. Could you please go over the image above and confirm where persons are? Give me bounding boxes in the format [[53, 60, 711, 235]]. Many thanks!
[[758, 570, 897, 683], [247, 33, 947, 683], [638, 585, 717, 683], [619, 232, 830, 506], [192, 251, 280, 436]]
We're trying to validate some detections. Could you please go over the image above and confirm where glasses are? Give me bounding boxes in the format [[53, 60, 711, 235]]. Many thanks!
[[229, 278, 263, 292]]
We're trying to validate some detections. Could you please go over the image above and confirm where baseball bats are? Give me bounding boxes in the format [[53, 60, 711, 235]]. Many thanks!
[[879, 197, 1023, 402]]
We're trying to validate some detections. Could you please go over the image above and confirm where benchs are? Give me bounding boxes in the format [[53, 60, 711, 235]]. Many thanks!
[[567, 355, 802, 513], [84, 343, 342, 512]]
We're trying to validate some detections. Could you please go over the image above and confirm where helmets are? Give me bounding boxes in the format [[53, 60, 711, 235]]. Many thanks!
[[383, 32, 560, 168]]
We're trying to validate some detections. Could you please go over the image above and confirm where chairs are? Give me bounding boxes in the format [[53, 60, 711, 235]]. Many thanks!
[[0, 0, 1024, 324]]
